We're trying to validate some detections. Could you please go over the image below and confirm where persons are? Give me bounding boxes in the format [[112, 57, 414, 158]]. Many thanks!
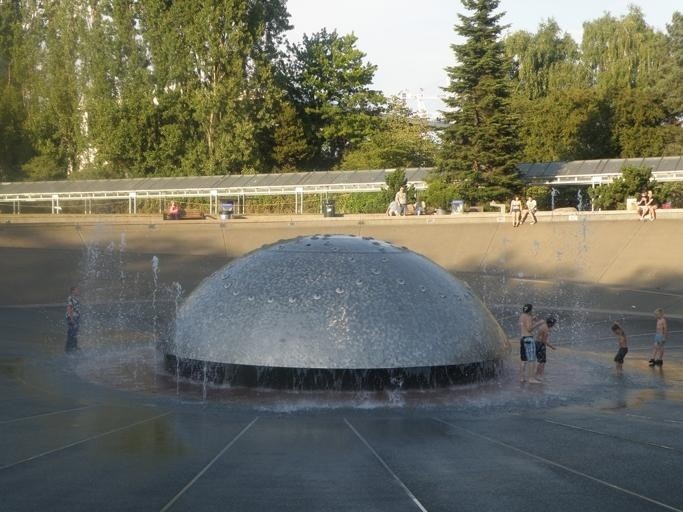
[[507, 194, 522, 227], [635, 189, 648, 221], [393, 185, 407, 217], [535, 317, 556, 381], [611, 323, 628, 374], [647, 190, 659, 221], [167, 200, 180, 220], [647, 307, 667, 366], [62, 285, 82, 355], [518, 303, 542, 383], [519, 194, 537, 225]]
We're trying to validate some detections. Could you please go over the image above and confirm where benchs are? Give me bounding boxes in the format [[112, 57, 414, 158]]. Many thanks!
[[402, 204, 425, 215], [163, 208, 205, 220]]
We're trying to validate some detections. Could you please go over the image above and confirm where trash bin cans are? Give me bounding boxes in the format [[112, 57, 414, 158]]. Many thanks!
[[219, 211, 230, 219], [450, 200, 463, 215], [324, 199, 335, 217]]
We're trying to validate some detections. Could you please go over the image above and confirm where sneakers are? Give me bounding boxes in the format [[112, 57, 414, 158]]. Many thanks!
[[648, 358, 664, 364]]
